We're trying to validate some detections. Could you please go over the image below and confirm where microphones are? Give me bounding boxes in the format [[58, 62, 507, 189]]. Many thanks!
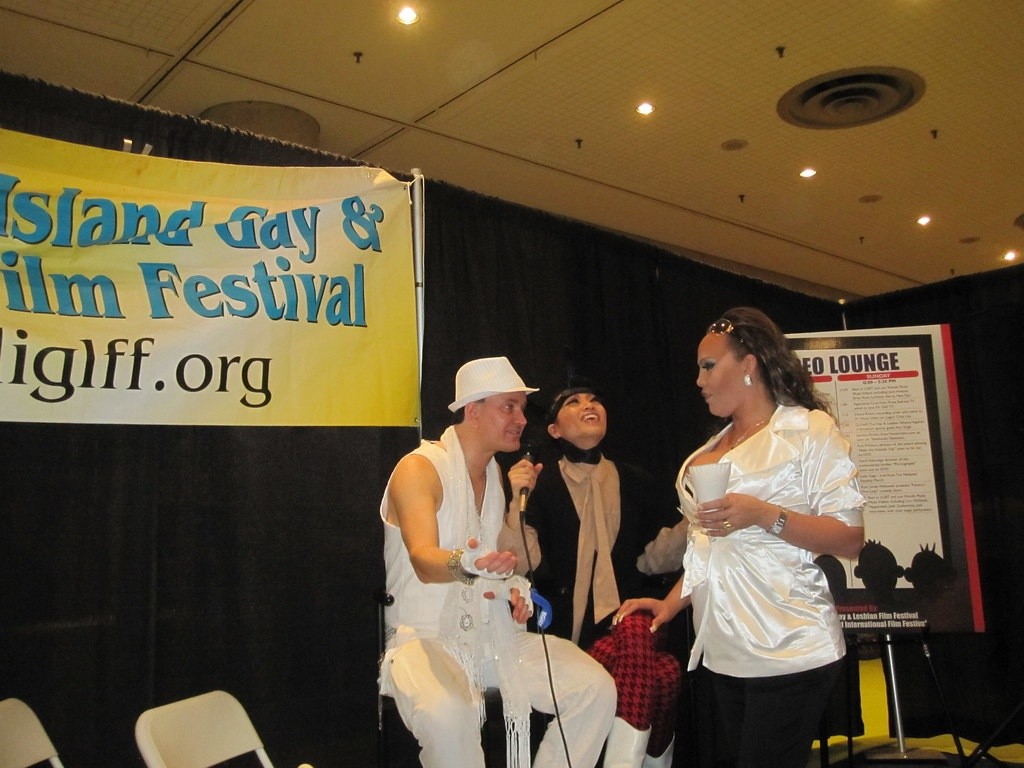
[[519, 440, 537, 521]]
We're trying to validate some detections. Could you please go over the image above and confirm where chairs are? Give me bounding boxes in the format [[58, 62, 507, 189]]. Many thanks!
[[0, 698, 65, 768], [134, 690, 278, 768]]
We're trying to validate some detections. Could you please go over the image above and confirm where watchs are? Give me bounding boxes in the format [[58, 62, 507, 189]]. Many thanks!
[[769, 505, 788, 537]]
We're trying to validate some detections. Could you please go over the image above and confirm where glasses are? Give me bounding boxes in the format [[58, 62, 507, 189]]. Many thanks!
[[707, 319, 752, 337]]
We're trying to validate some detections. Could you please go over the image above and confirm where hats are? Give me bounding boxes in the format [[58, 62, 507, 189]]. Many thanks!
[[447, 356, 541, 415]]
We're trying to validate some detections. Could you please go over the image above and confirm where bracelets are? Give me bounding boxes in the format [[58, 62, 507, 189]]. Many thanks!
[[449, 549, 475, 586]]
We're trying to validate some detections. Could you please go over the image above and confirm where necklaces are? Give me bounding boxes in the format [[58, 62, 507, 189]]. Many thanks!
[[720, 419, 768, 454]]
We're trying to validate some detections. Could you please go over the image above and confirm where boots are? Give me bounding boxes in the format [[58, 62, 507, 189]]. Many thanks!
[[601, 716, 675, 767]]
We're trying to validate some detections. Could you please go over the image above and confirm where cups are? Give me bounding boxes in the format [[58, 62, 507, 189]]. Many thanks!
[[688, 461, 732, 533]]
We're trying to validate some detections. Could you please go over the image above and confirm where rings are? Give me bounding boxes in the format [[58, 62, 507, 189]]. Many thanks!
[[723, 520, 734, 531]]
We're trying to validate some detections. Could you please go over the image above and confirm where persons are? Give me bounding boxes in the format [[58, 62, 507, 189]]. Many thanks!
[[379, 356, 617, 768], [497, 385, 679, 767], [611, 306, 867, 767]]
